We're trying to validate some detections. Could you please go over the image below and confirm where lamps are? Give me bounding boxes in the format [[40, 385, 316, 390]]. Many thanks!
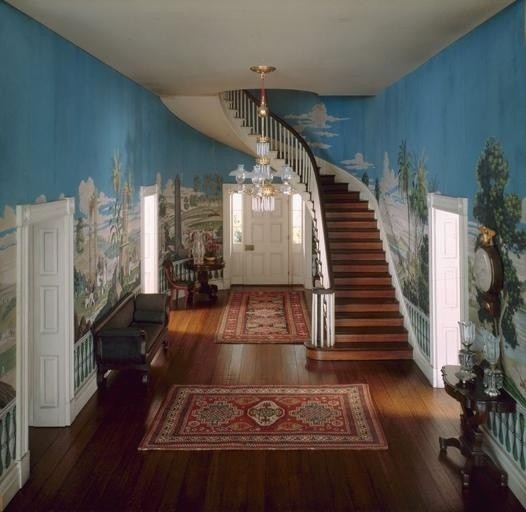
[[227, 66, 297, 213]]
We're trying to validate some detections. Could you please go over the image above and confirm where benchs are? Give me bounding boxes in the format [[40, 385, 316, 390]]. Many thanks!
[[90, 291, 174, 386]]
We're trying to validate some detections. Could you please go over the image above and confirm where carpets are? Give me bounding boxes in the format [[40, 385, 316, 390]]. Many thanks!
[[138, 383, 390, 452], [214, 283, 315, 344]]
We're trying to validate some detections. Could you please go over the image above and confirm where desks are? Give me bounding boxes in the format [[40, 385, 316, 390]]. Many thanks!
[[186, 262, 226, 295], [440, 364, 518, 489]]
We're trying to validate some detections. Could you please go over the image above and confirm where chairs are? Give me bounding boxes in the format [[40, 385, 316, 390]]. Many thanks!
[[162, 259, 195, 310]]
